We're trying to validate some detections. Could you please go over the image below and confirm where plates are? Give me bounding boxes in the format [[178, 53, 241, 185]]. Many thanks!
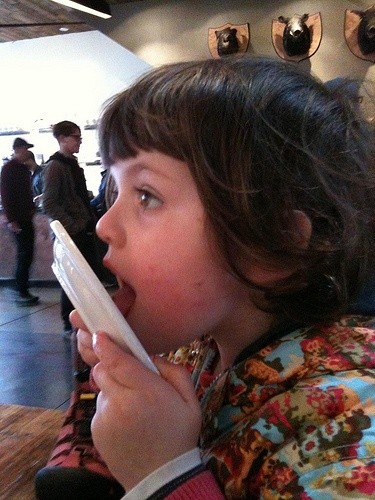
[[50, 220, 160, 375]]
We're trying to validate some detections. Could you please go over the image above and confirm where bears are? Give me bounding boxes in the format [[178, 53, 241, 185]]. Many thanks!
[[214, 27, 240, 58], [350, 3, 375, 56], [278, 14, 313, 57]]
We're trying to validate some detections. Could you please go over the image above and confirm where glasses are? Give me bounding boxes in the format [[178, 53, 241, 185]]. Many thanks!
[[64, 134, 83, 142]]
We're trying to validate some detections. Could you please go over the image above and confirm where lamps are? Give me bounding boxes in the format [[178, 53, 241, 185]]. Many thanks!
[[51, 0, 112, 19]]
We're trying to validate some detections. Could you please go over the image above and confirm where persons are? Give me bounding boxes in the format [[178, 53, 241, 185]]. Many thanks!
[[0, 138, 40, 307], [69, 54, 375, 500], [42, 121, 98, 333], [25, 151, 44, 207], [90, 151, 117, 288]]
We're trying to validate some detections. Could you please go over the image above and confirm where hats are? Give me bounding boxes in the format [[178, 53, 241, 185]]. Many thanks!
[[12, 137, 34, 149]]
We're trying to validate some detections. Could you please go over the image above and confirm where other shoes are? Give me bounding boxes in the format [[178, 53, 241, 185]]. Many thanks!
[[14, 292, 40, 306]]
